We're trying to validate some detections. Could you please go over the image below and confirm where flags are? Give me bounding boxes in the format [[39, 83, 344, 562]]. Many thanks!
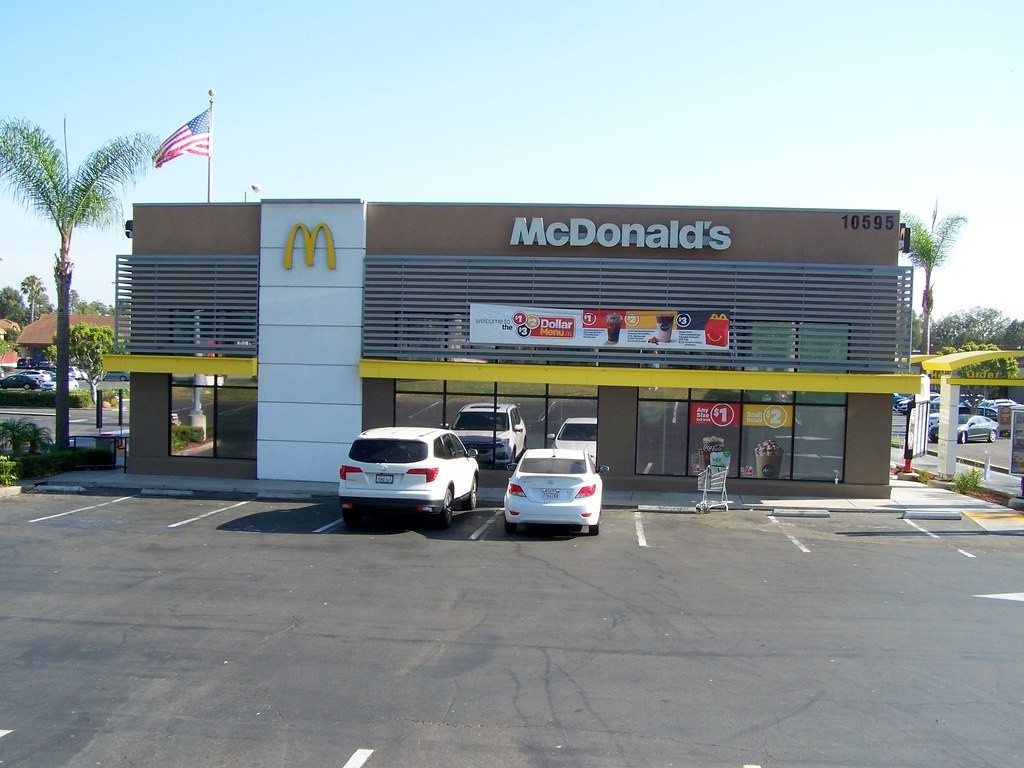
[[151, 107, 210, 168]]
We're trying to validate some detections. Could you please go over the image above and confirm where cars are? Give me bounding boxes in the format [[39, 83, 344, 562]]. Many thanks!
[[91, 370, 130, 381], [893, 392, 1017, 417], [503, 449, 610, 535], [0, 357, 93, 390], [547, 418, 597, 467], [42, 376, 79, 392], [929, 414, 1001, 444]]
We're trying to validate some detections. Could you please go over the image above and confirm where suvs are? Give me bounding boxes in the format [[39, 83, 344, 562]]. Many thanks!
[[338, 427, 480, 531], [440, 403, 527, 464]]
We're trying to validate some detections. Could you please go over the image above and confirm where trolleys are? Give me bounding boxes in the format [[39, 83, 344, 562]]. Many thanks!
[[695, 464, 730, 514]]
